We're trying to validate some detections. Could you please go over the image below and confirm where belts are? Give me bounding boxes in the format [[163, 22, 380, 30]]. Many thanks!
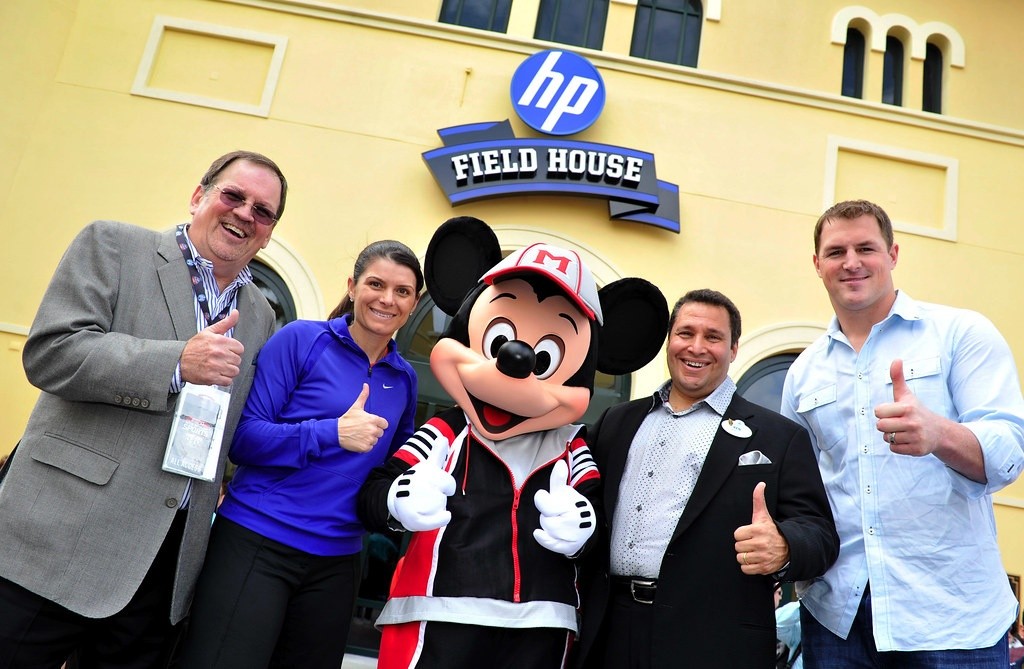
[[610, 580, 657, 604]]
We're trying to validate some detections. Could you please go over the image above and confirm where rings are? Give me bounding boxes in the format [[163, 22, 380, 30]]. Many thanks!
[[888, 433, 896, 444], [743, 552, 749, 565]]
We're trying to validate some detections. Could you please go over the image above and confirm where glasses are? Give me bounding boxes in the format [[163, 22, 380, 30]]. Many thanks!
[[211, 182, 278, 226]]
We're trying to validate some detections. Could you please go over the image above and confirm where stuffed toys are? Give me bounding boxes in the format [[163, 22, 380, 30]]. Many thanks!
[[355, 216, 670, 669]]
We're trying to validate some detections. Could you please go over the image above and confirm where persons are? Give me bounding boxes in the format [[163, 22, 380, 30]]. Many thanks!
[[779, 200, 1024, 669], [0, 151, 287, 669], [170, 240, 423, 669], [585, 290, 841, 669]]
